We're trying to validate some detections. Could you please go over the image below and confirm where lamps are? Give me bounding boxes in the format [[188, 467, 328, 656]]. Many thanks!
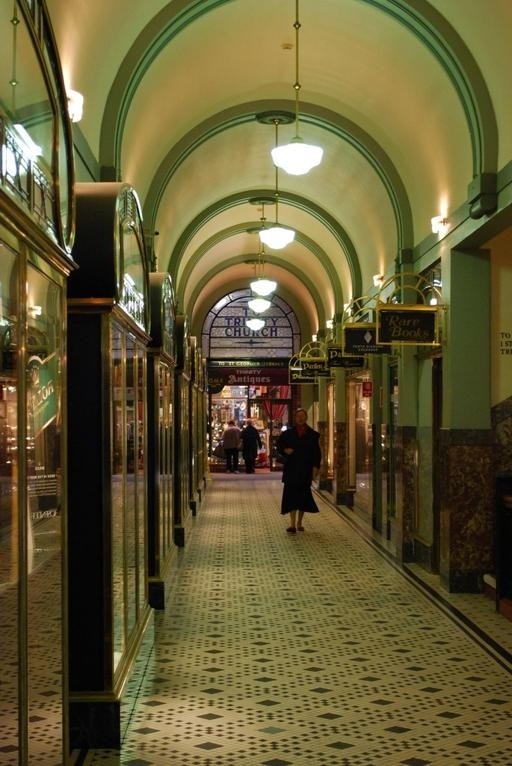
[[245, 260, 266, 332], [254, 109, 296, 250], [248, 227, 272, 313], [271, 1, 324, 176], [250, 196, 278, 295]]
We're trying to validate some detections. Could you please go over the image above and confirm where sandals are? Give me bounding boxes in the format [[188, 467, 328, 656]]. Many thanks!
[[286, 526, 305, 533]]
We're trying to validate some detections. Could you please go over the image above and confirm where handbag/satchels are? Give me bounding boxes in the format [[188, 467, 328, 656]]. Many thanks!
[[277, 448, 289, 464]]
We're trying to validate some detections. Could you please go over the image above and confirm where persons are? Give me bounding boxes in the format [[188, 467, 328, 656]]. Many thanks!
[[239, 419, 263, 474], [275, 406, 323, 534], [221, 419, 242, 474]]
